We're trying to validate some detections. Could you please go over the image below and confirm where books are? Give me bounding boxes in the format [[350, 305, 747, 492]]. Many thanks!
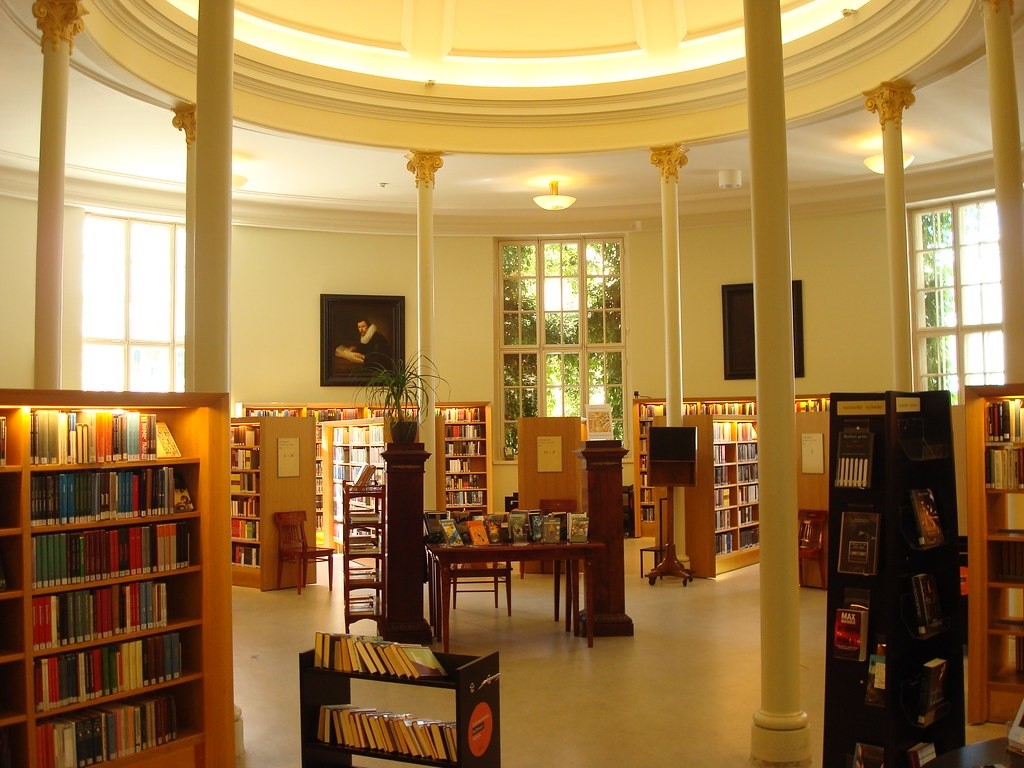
[[684, 402, 759, 553], [333, 426, 384, 540], [36, 692, 177, 768], [796, 398, 830, 412], [0, 410, 182, 467], [34, 631, 182, 711], [424, 510, 589, 547], [31, 520, 189, 590], [832, 428, 946, 768], [247, 408, 298, 416], [799, 520, 821, 549], [986, 401, 1024, 672], [231, 425, 260, 566], [436, 408, 483, 504], [639, 402, 663, 521], [371, 408, 416, 417], [307, 408, 360, 528], [315, 631, 448, 679], [318, 704, 457, 762], [31, 465, 195, 525], [32, 582, 166, 651]]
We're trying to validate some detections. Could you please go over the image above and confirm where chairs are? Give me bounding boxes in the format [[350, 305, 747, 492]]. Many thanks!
[[449, 561, 513, 616], [798, 509, 827, 591], [639, 497, 669, 581], [274, 511, 335, 595], [520, 499, 576, 579]]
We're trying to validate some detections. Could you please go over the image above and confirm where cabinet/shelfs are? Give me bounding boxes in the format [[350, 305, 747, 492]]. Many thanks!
[[632, 393, 830, 538], [341, 480, 387, 636], [517, 417, 589, 573], [243, 401, 493, 547], [322, 416, 446, 572], [653, 415, 758, 578], [822, 391, 966, 768], [230, 416, 316, 592], [299, 649, 501, 768], [0, 388, 235, 768], [965, 384, 1024, 725]]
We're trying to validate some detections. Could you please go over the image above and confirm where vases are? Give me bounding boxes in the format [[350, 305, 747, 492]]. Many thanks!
[[349, 350, 451, 442]]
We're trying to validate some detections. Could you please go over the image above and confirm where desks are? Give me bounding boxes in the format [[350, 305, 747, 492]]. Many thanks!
[[427, 543, 605, 653]]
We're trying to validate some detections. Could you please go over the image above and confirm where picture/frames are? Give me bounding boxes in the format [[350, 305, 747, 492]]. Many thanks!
[[319, 294, 405, 386], [722, 280, 804, 380]]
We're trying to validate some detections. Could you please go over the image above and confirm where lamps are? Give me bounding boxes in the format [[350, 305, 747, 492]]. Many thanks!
[[864, 152, 914, 174], [533, 181, 576, 210]]
